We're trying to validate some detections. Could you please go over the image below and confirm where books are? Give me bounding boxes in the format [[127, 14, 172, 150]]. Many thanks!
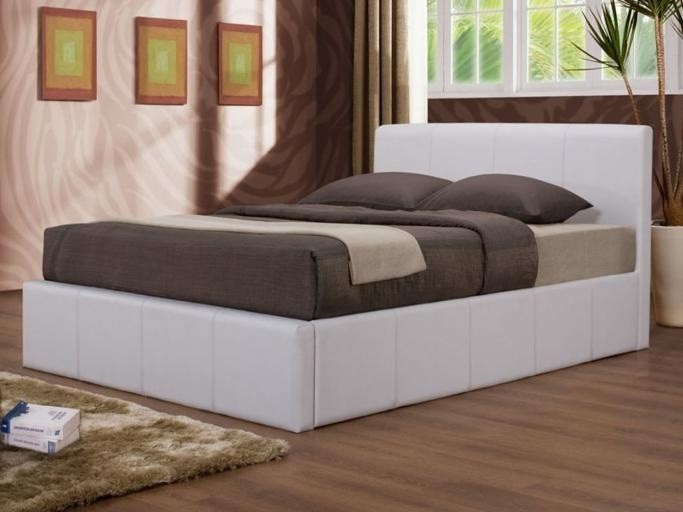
[[0, 399, 80, 441], [0, 424, 81, 455]]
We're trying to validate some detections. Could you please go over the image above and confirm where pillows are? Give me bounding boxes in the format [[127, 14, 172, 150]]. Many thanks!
[[296, 173, 594, 225]]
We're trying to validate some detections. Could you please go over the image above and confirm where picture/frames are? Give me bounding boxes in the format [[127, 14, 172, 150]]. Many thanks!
[[133, 16, 188, 106], [216, 21, 262, 106], [38, 7, 97, 102]]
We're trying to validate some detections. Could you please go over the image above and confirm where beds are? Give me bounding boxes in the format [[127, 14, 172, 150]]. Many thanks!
[[21, 121, 655, 433]]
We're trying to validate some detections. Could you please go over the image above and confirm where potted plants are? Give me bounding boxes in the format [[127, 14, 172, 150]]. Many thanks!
[[564, 1, 683, 328]]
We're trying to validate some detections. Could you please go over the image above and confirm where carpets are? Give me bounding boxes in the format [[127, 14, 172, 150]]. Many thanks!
[[0, 369, 291, 510]]
